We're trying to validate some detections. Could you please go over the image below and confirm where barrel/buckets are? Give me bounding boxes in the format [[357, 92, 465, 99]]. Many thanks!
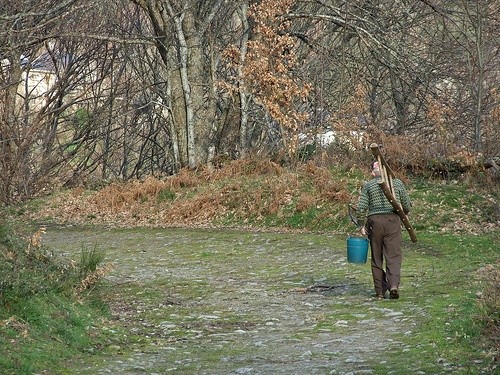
[[346, 237, 370, 264]]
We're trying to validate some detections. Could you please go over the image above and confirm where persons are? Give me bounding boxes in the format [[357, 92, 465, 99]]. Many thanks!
[[356, 162, 410, 299]]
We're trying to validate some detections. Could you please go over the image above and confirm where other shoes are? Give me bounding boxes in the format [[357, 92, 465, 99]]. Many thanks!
[[378, 294, 386, 299], [388, 288, 400, 299]]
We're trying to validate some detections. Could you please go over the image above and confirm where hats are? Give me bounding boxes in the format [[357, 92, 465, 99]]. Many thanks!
[[372, 162, 379, 169]]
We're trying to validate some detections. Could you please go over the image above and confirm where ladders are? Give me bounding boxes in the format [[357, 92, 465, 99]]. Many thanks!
[[370, 143, 418, 243]]
